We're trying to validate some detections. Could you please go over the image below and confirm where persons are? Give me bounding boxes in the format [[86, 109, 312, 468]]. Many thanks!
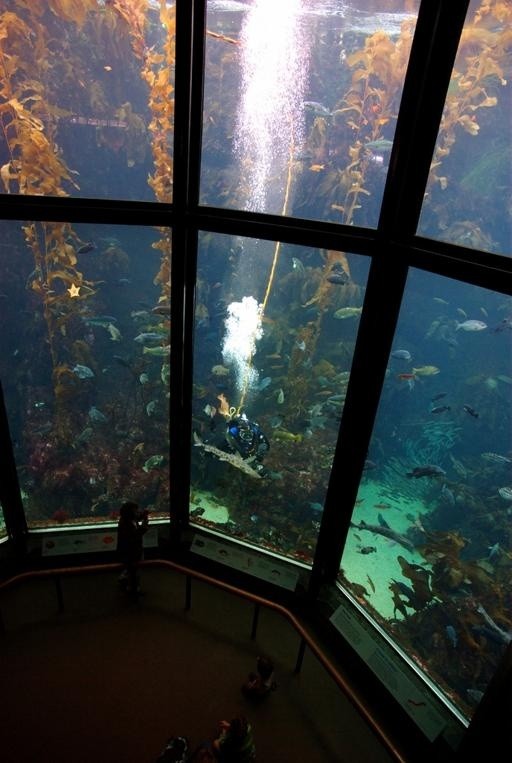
[[237, 416, 271, 468], [227, 419, 274, 483], [152, 652, 287, 763], [116, 502, 151, 586]]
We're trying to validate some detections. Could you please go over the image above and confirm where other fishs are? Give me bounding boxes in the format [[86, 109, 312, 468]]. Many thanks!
[[192, 255, 363, 489], [346, 296, 512, 647]]
